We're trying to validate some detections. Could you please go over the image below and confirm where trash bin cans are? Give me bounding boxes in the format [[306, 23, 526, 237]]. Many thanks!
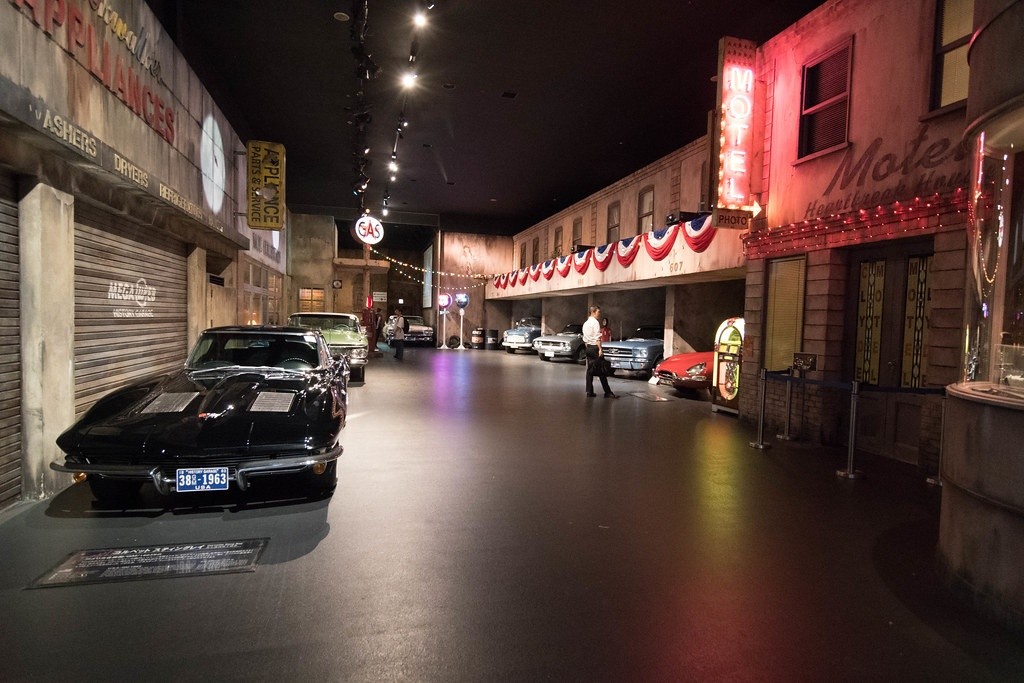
[[486, 328, 498, 350], [471, 327, 486, 349]]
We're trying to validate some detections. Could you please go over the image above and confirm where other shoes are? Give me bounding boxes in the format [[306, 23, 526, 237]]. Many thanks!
[[393, 355, 403, 360], [604, 392, 620, 398], [587, 392, 596, 397]]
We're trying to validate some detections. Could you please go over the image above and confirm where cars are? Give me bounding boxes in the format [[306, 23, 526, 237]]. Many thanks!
[[285, 310, 369, 380], [502, 317, 541, 354], [649, 351, 714, 398], [57, 325, 355, 507], [600, 325, 662, 380], [533, 323, 587, 363], [382, 313, 435, 347]]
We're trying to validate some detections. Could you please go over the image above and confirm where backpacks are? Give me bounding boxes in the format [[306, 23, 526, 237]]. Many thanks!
[[397, 316, 409, 334]]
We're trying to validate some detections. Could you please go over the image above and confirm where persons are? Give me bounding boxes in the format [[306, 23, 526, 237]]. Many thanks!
[[374, 308, 383, 348], [393, 307, 406, 360], [599, 318, 612, 342], [581, 306, 622, 399]]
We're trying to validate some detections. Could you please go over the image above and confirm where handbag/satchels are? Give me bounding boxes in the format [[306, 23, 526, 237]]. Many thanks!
[[588, 356, 614, 377]]
